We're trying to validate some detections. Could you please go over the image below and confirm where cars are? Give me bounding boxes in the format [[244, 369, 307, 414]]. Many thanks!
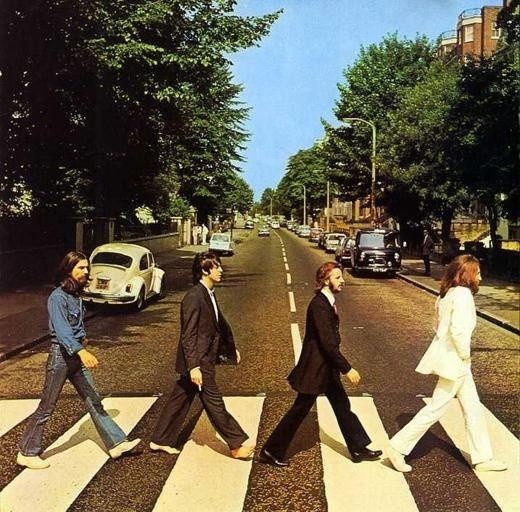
[[324, 232, 347, 252], [350, 226, 407, 275], [81, 243, 167, 311], [244, 214, 329, 247], [335, 237, 356, 263]]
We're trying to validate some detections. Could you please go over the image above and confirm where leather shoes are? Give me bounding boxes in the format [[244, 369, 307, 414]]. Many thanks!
[[387, 448, 412, 472], [475, 460, 508, 471], [16, 452, 50, 469], [351, 448, 382, 463], [259, 448, 290, 467], [109, 438, 141, 459]]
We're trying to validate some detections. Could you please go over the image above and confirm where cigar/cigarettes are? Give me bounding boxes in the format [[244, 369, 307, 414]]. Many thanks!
[[198, 383, 202, 391]]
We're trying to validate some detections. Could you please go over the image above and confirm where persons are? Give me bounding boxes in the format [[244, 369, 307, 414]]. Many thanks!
[[201, 223, 209, 246], [16, 251, 143, 469], [421, 229, 433, 275], [259, 262, 383, 467], [149, 253, 254, 460], [379, 255, 510, 471]]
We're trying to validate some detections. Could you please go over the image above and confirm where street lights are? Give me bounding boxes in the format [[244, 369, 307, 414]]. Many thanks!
[[294, 183, 306, 225], [343, 117, 376, 220], [313, 169, 329, 213]]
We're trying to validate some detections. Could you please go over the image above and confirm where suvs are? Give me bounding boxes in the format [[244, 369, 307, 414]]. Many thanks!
[[209, 233, 236, 255]]
